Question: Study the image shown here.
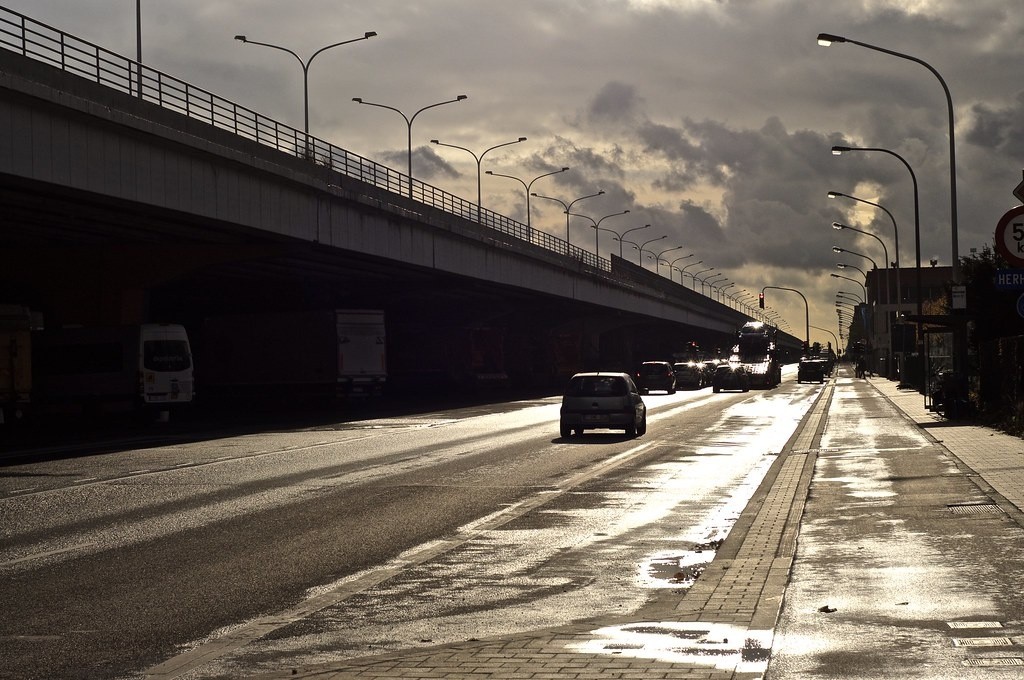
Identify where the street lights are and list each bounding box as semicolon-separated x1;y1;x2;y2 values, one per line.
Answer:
591;224;652;258
633;244;682;277
531;190;607;255
660;261;705;285
825;189;906;389
831;246;881;376
352;91;467;200
232;28;377;164
612;235;667;268
816;31;961;287
713;285;735;305
486;165;569;243
432;136;527;222
705;282;734;303
674;267;714;293
832;217;893;381
686;271;722;295
695;278;729;299
726;290;795;337
649;252;696;283
830;144;928;395
568;210;632;268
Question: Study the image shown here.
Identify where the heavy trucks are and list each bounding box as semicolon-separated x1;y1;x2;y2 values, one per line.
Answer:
729;336;782;389
190;305;390;406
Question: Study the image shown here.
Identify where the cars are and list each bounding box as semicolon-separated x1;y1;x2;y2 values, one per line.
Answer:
712;364;750;392
737;320;769;343
557;370;645;438
796;360;824;386
638;361;678;395
694;362;712;389
673;362;702;390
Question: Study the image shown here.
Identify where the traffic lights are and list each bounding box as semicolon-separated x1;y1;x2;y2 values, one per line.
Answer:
758;293;765;310
691;339;697;349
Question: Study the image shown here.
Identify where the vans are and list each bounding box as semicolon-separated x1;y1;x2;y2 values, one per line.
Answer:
38;323;194;423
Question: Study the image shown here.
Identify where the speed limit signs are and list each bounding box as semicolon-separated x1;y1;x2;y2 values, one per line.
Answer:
993;203;1024;269
